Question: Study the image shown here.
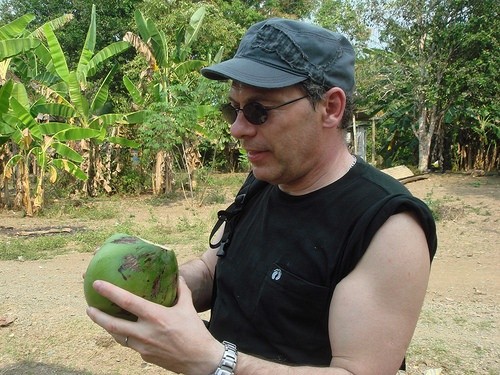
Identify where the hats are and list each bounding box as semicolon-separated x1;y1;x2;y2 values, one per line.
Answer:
202;18;356;96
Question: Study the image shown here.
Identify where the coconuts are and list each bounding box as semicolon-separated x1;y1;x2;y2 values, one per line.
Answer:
83;233;179;322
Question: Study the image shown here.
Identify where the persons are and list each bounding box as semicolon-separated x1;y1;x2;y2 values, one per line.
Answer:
82;17;438;375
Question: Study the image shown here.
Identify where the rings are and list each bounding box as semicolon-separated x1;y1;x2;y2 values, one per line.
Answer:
125;336;128;347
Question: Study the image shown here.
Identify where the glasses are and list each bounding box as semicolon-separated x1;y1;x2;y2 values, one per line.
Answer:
221;95;315;125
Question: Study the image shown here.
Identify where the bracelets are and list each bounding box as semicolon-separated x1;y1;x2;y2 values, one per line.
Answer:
210;341;238;375
